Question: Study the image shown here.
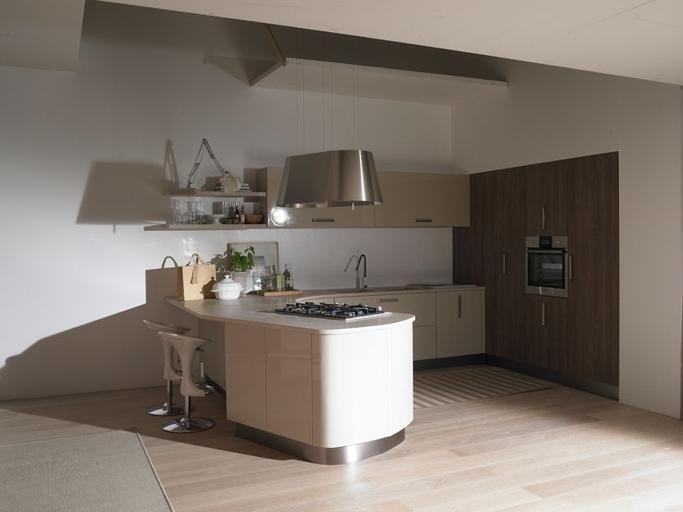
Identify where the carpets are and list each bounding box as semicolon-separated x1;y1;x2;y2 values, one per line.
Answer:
409;365;555;410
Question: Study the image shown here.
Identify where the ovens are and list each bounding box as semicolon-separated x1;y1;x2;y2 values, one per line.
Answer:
522;235;572;300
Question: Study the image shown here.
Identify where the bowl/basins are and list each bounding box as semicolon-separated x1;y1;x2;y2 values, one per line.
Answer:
245;214;264;224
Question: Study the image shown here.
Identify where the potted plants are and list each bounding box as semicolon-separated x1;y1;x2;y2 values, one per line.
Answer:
243;246;255;292
229;246;249;295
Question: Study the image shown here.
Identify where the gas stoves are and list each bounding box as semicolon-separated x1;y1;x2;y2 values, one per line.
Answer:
277;299;389;322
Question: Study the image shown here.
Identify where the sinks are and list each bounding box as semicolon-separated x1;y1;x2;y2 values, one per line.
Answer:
340;288;375;294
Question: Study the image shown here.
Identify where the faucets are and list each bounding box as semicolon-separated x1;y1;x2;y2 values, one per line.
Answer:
343;255;360;289
355;253;367;289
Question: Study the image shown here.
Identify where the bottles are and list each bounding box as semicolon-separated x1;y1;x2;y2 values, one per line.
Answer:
234;206;245;224
284;264;291;292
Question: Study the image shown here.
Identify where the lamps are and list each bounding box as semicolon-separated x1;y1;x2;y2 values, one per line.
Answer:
273;60;382;212
180;135;242;195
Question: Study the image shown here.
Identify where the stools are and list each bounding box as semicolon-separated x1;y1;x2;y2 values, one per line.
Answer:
141;318;217;434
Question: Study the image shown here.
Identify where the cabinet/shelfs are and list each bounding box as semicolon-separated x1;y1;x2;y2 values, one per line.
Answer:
562;152;619;401
525;295;566;372
525;162;561;235
162;190;267;229
372;170;472;229
434;291;485;359
334;293;436;361
256;166;374;228
451;167;526;364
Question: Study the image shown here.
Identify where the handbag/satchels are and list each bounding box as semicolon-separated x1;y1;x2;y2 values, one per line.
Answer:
175;253;217;301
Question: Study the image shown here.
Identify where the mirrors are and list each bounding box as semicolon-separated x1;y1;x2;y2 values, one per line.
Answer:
227;241;280;295
